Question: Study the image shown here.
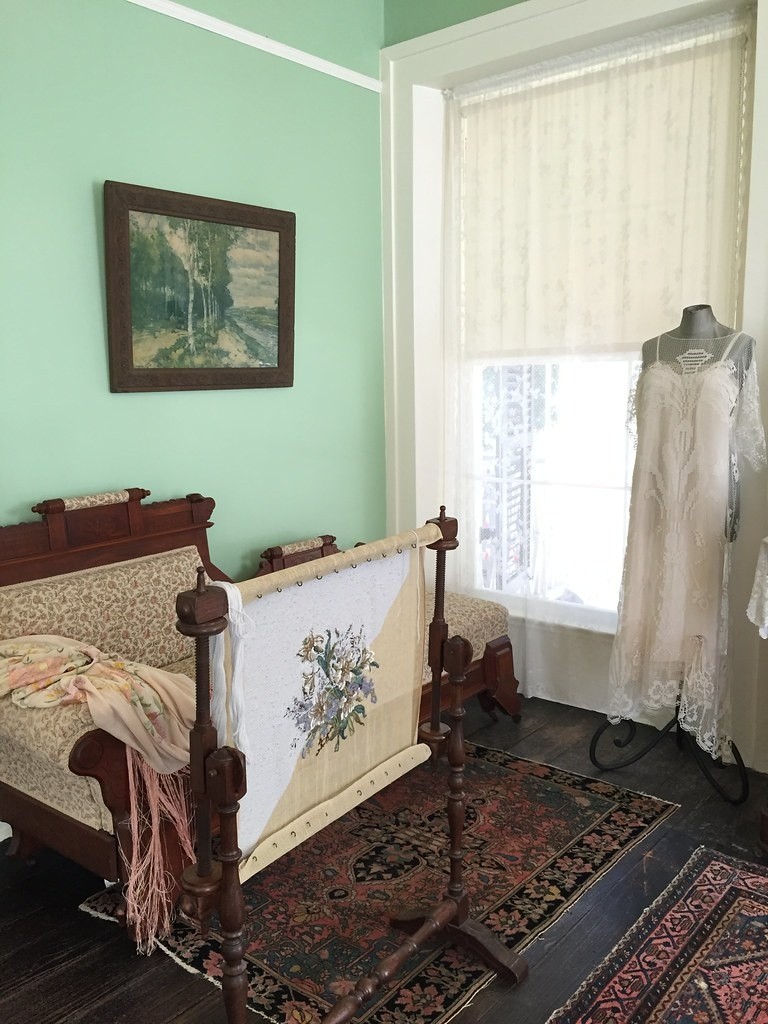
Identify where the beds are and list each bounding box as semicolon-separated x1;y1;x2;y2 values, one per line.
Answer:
0;487;529;1023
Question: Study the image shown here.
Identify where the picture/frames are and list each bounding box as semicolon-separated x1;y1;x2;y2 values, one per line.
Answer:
104;180;296;393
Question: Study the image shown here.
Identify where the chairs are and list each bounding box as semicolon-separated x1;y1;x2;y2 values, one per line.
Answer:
255;535;522;725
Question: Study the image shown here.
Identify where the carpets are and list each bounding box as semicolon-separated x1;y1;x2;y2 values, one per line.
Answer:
546;845;768;1024
80;739;681;1024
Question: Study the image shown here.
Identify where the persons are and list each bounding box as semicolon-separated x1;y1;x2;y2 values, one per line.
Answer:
609;304;765;764
